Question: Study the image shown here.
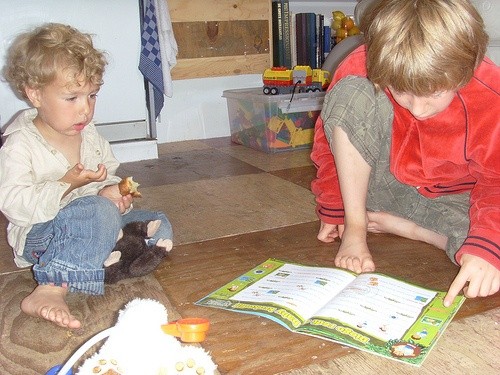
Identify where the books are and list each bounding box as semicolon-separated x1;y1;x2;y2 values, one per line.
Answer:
272;0;333;69
195;257;466;367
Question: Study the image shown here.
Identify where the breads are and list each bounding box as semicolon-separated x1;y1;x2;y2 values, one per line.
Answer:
118;175;142;198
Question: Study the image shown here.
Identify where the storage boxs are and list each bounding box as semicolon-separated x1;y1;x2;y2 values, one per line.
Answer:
221;87;326;153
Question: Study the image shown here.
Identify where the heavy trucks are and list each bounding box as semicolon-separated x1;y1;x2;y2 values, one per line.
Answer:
261;65;332;95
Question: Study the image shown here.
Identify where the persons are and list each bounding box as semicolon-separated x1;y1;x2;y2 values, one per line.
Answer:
310;0;500;306
0;21;173;330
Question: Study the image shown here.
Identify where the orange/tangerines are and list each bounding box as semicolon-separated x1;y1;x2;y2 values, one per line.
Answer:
332;11;360;43
